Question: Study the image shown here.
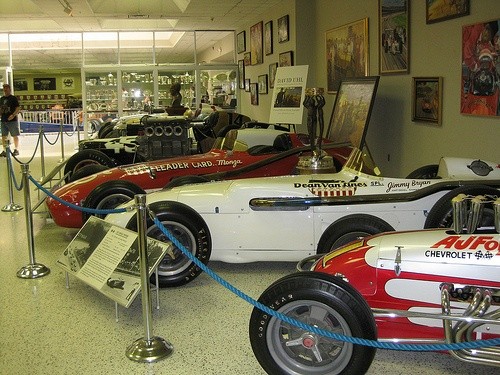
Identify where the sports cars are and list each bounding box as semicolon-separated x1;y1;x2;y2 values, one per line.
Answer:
104;146;500;290
44;127;360;243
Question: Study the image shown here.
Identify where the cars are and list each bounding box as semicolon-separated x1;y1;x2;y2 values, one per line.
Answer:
64;107;288;185
247;192;500;375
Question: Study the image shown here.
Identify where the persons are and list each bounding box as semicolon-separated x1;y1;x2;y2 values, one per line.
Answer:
302;94;326;138
169;81;182;107
0;84;20;157
275;88;285;107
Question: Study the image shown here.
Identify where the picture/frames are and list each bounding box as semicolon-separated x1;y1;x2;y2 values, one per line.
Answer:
250;21;263;65
249;83;258;105
279;51;293;67
245;79;250;92
277;15;289;43
326;76;381;158
237;31;246;54
265;20;273;56
238;60;245;89
325;17;370;93
426;0;470;25
258;74;268;94
378;0;410;75
269;62;278;88
244;52;251;65
412;76;442;125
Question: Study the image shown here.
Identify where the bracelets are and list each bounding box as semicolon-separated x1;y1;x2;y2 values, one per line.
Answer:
12;114;15;117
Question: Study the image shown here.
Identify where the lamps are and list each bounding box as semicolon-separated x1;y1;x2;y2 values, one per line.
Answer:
64;8;72;15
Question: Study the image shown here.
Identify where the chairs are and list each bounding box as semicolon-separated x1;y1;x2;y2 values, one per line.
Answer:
273;133;291;151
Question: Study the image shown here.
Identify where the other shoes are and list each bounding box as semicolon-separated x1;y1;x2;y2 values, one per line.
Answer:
13;149;19;155
0;151;6;157
